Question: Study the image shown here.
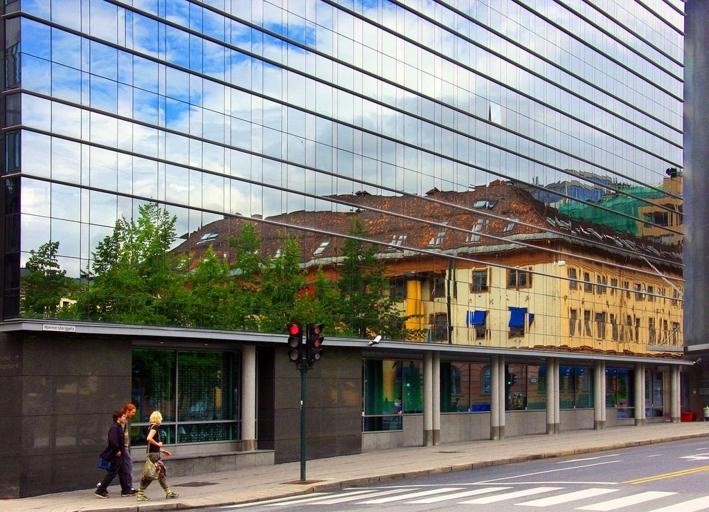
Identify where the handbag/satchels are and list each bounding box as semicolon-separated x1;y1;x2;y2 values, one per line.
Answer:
100;447;117;460
144;457;160;480
97;458;113;471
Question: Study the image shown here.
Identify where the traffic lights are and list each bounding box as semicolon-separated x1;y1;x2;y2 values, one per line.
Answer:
306;322;327;361
285;323;303;363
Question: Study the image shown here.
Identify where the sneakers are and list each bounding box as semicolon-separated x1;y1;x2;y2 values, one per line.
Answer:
95;489;110;499
121;490;137;497
138;495;150;502
166;492;179;499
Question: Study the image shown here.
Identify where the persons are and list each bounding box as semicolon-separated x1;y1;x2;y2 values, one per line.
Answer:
94;409;137;497
136;410;179;501
95;403;139;493
